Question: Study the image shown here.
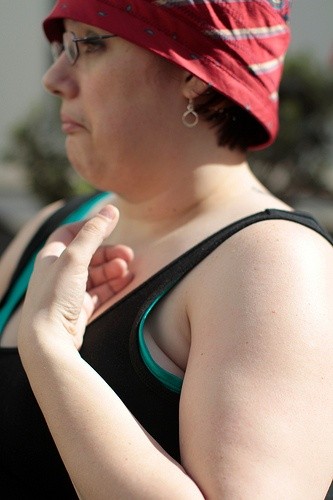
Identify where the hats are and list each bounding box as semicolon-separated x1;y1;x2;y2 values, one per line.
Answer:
43;0;290;151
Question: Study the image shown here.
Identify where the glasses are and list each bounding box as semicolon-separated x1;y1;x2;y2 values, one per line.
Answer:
51;32;118;65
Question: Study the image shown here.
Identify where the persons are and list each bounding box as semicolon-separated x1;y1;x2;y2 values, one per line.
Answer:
1;0;333;500
